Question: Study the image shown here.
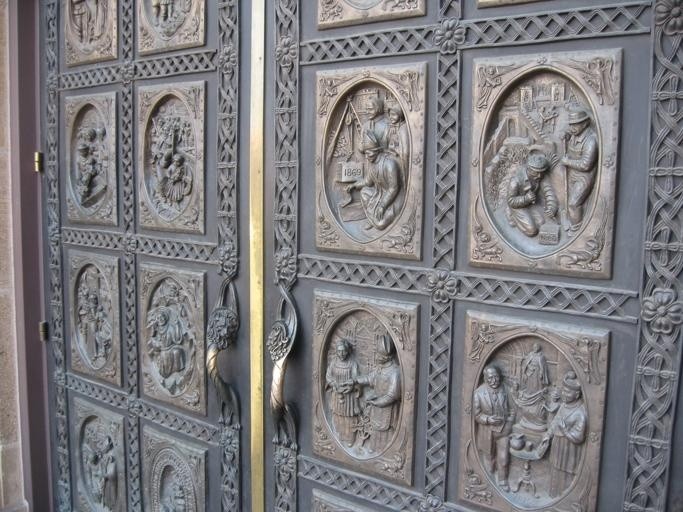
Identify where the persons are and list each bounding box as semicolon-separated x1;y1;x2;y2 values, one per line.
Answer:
76;1;194;512
325;100;600;512
